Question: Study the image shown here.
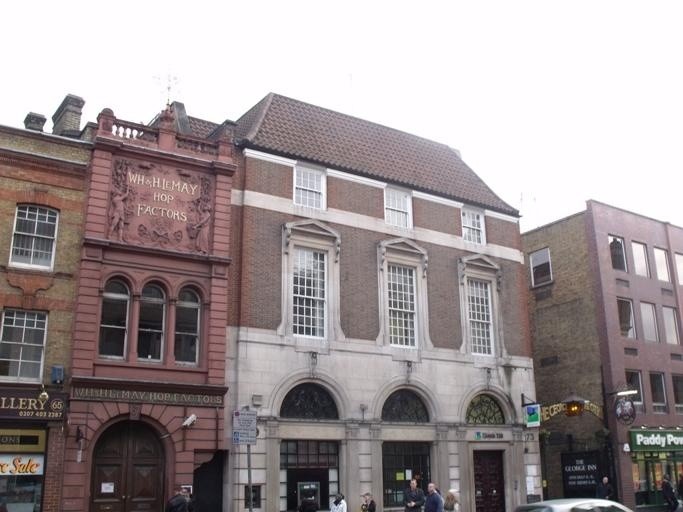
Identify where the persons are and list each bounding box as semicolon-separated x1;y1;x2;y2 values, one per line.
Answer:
402;478;425;512
165;485;188;512
183;488;200;512
329;493;348;512
660;473;679;512
424;482;444;512
298;491;320;512
106;182;131;245
191;199;212;256
595;476;615;500
444;493;462;512
362;492;376;512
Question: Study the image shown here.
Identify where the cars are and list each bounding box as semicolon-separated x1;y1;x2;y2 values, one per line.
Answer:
513;496;636;512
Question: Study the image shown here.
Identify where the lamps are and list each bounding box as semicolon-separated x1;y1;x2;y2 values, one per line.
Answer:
623;443;631;452
38;384;48;400
